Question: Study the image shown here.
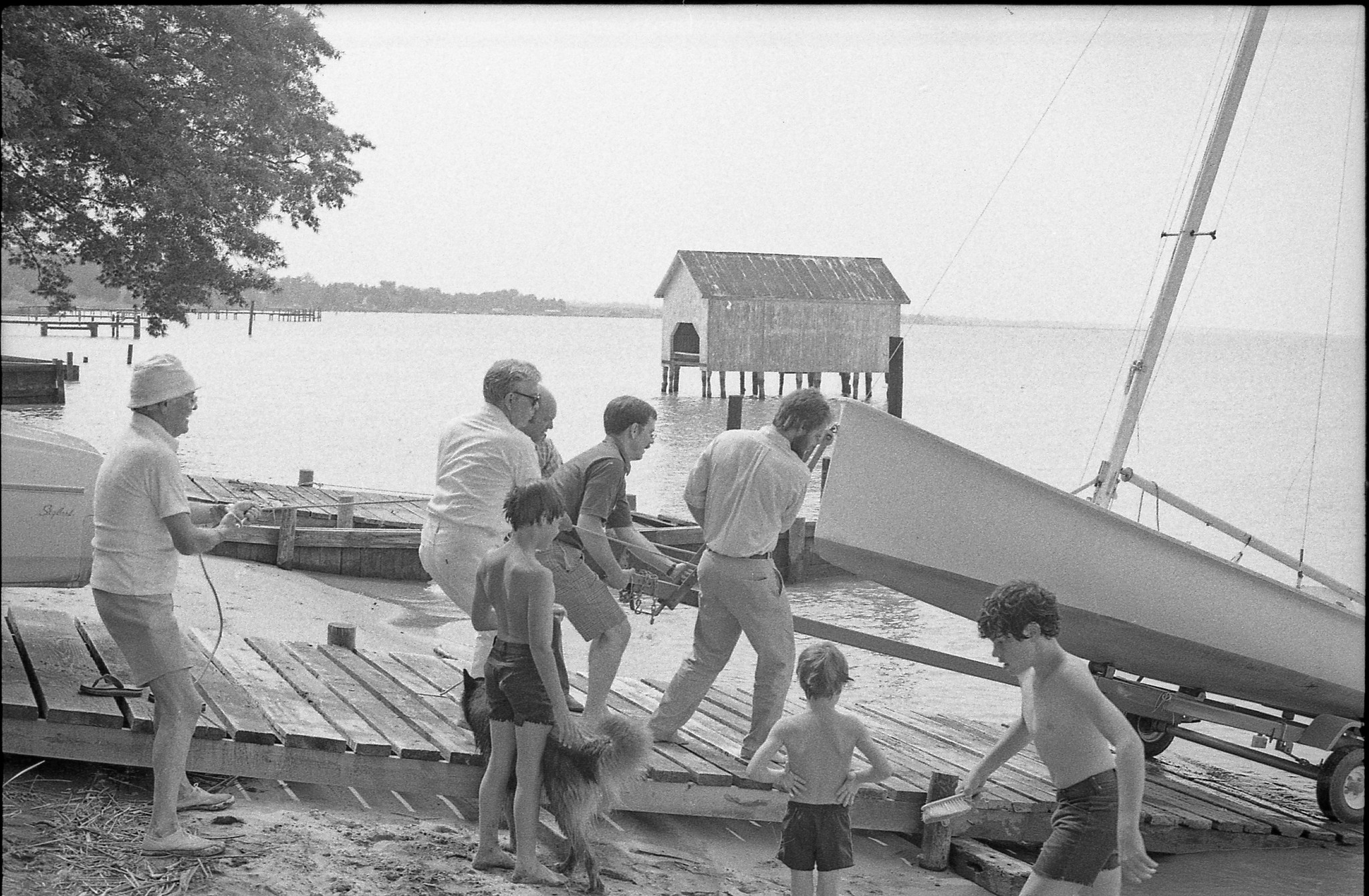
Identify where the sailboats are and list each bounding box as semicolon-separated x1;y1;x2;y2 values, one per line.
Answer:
806;6;1366;730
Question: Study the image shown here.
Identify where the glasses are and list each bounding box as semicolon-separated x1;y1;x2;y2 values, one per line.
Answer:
504;391;540;406
639;425;657;439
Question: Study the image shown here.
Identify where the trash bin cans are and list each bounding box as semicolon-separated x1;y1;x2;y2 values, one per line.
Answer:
1;418;104;587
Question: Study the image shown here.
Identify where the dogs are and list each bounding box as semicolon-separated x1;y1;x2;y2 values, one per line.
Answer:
460;666;658;896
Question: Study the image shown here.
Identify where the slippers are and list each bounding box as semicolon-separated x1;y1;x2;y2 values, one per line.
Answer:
80;675;142;697
147;692;207;711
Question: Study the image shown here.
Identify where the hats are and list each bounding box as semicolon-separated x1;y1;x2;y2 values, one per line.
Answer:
127;352;196;409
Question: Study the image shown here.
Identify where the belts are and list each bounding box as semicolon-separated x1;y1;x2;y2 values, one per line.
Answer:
706;546;773;559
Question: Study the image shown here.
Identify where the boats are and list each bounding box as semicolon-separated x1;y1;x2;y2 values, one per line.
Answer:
184;471;435;580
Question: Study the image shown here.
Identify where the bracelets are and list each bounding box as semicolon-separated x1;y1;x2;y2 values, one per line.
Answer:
666;562;677;576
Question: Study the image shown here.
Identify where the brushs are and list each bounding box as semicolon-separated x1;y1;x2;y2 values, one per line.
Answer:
920;787;990;824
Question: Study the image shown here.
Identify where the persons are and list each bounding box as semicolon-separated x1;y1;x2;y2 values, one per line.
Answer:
417;358;546;706
89;360;262;856
645;387;835;763
469;480;584;887
522;384;585;713
536;396;694;740
953;580;1160;896
740;640;894;896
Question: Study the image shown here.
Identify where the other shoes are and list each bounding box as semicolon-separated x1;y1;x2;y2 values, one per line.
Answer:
176;783;235;811
564;694;584;712
142;825;225;856
